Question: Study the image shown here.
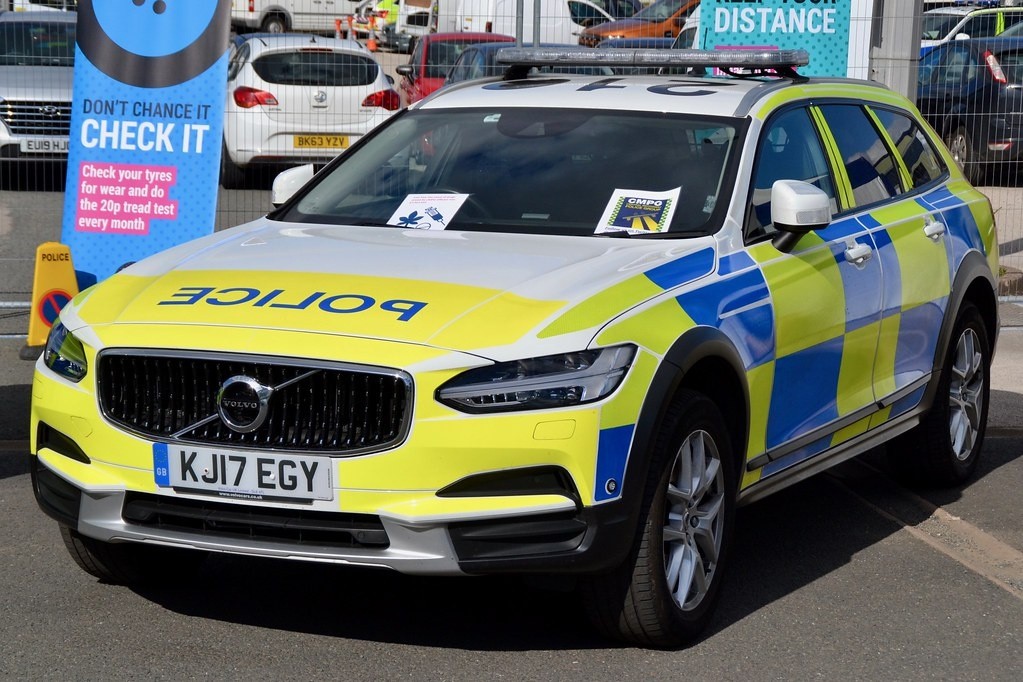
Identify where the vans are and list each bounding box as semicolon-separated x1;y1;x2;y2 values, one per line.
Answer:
455;0;615;54
396;0;457;49
231;1;362;34
658;4;701;75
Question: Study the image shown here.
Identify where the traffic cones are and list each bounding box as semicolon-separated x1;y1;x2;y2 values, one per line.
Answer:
336;16;380;52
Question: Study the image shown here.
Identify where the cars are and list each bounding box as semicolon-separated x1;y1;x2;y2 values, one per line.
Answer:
396;34;529;164
352;0;429;50
919;6;1023;184
31;50;1002;648
222;36;405;189
578;1;700;55
0;10;77;172
